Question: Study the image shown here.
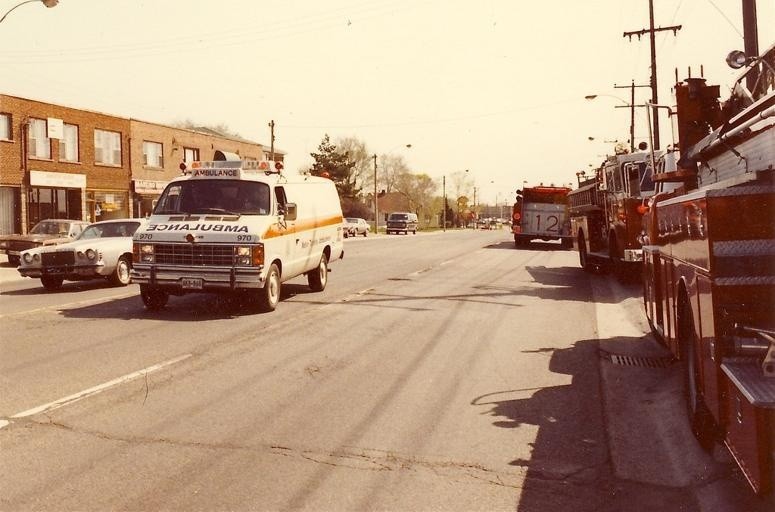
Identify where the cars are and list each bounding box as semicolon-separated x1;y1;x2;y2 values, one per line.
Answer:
346;217;371;237
0;219;93;264
342;218;350;239
19;218;148;288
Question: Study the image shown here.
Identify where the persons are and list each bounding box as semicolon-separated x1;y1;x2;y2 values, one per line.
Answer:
183;185;210;210
246;184;269;214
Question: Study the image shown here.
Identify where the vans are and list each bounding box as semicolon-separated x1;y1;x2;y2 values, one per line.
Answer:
386;212;419;234
129;159;345;309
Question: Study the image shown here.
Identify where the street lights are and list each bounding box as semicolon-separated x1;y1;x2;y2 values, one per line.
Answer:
0;0;59;23
